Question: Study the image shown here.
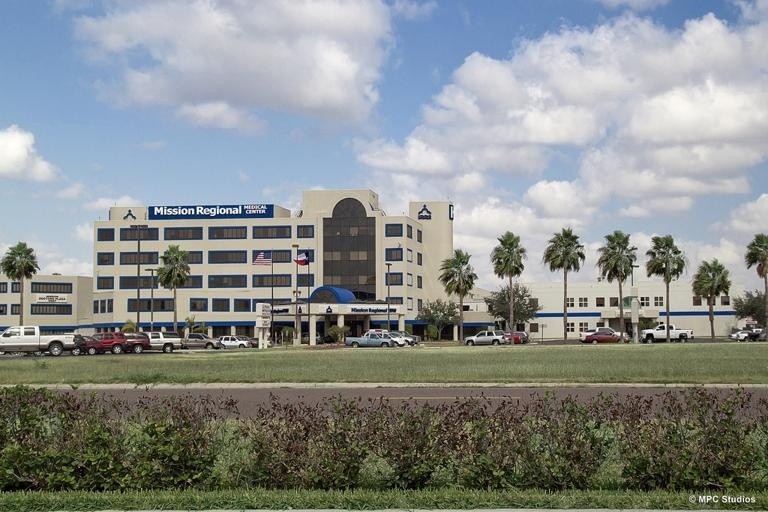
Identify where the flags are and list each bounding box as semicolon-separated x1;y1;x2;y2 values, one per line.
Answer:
252;251;272;266
293;250;313;266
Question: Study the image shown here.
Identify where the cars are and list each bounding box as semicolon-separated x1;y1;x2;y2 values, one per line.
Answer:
71;331;273;356
728;328;768;342
464;330;530;346
578;327;631;344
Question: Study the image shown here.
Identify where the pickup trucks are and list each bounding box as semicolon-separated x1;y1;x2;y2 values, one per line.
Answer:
0;325;75;357
638;324;694;344
345;328;422;348
